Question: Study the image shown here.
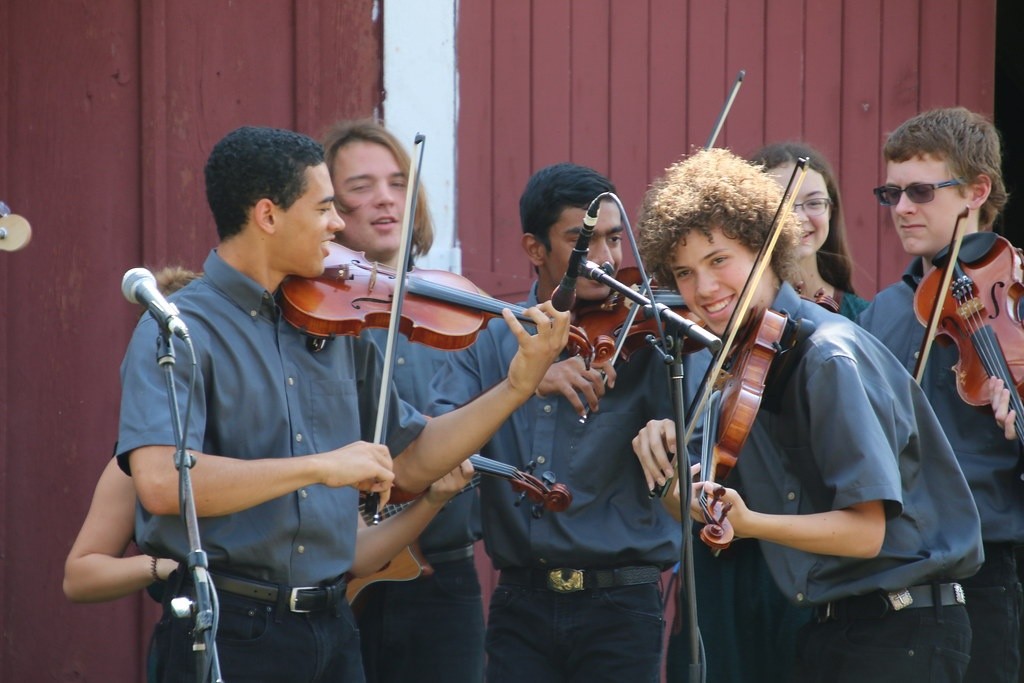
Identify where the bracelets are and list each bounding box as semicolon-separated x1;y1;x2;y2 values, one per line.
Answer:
535;388;548;400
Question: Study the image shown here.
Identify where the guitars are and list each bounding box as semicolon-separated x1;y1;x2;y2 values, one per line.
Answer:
344;468;487;608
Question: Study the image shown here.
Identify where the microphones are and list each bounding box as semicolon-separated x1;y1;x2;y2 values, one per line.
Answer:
121;267;190;340
552;200;600;312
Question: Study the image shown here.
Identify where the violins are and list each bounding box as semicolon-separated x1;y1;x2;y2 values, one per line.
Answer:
421;412;573;521
567;264;842;373
278;237;618;373
696;304;789;560
909;230;1024;483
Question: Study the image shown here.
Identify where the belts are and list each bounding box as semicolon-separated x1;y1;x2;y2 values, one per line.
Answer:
814;583;966;624
207;571;347;613
498;565;661;593
425;546;472;566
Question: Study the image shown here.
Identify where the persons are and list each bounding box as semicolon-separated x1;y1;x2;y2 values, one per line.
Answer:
117;120;571;683
633;143;990;683
63;122;880;683
857;99;1024;683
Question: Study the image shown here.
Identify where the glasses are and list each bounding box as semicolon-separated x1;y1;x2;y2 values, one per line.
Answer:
873;176;973;206
789;197;833;217
